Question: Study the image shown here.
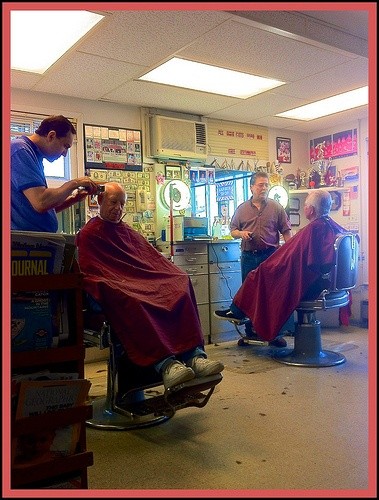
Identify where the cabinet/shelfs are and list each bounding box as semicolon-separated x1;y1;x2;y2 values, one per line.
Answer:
156;240;247;346
12;258;93;489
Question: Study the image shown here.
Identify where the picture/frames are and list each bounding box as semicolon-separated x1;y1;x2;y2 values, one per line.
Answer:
290;213;300;226
289;198;300;211
276;136;291;163
164;165;183;180
330;191;341;211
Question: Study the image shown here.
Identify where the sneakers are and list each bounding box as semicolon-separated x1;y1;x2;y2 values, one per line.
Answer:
182;355;224;377
214;309;241;321
161;360;195;388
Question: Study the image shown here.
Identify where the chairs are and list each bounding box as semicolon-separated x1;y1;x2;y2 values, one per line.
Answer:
230;233;360;368
80;290;222;431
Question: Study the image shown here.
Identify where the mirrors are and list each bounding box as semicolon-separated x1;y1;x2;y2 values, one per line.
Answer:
189;169;253;239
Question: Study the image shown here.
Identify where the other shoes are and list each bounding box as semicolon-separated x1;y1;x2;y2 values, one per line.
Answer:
238;338;249;346
269;338;287;347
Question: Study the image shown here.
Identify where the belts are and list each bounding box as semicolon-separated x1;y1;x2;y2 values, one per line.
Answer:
243;246;274;256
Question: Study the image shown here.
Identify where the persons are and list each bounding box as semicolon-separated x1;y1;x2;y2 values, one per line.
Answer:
77;181;224;386
215;189;350;341
230;171;292;348
10;115;100;232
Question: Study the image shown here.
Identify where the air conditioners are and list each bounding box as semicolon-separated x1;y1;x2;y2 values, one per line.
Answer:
150;116;208;159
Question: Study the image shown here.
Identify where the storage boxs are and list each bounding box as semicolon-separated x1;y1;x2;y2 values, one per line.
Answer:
166;217;209;242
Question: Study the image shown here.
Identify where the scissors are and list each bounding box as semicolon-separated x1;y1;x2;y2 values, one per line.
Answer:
248;233;253;241
